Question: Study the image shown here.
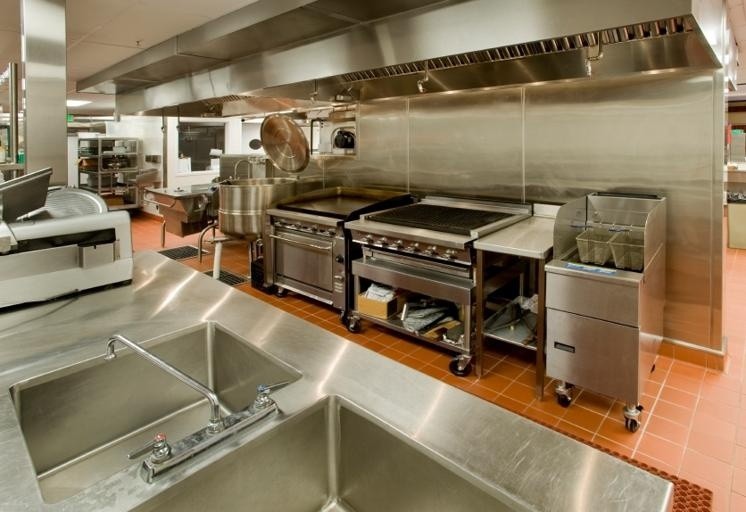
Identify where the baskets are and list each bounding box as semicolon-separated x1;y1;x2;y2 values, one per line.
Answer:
576;227;644;270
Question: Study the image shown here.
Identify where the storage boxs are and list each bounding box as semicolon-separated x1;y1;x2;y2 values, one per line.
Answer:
101;195;124;205
77;158;98;171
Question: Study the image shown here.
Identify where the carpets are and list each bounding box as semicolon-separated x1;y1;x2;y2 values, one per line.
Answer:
518;414;712;512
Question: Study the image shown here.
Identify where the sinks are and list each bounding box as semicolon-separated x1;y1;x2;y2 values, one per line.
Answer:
129;393;534;512
10;323;303;505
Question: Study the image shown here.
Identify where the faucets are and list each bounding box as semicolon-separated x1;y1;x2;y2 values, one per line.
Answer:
104;335;222;423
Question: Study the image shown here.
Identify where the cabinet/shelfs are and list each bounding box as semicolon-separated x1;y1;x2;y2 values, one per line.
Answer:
77;138;140;210
